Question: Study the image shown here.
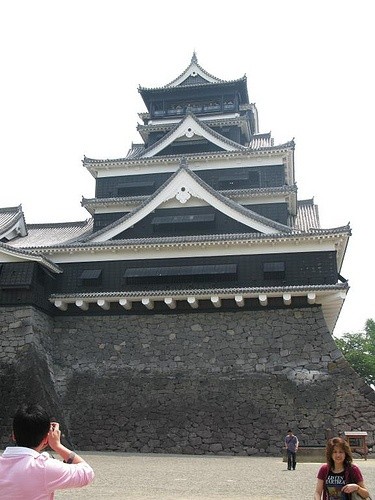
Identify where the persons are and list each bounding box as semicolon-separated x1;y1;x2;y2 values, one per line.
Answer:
313;438;371;500
0;402;94;500
284;429;299;470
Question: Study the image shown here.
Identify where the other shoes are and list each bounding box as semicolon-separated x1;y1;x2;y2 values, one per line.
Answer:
293;467;295;470
288;467;291;470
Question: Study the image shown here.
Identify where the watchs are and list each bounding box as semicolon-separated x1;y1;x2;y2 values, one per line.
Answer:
356;484;360;491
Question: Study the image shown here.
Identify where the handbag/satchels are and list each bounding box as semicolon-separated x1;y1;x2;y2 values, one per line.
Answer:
347;466;367;499
